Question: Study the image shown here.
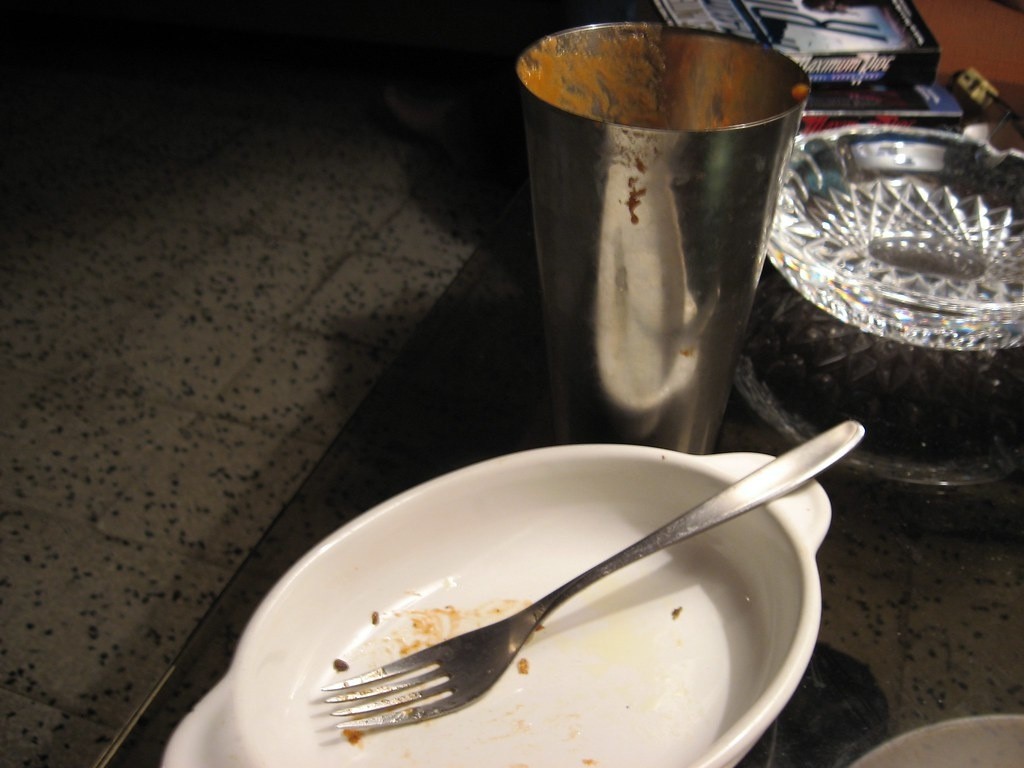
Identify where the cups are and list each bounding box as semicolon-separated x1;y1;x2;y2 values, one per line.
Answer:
513;20;811;460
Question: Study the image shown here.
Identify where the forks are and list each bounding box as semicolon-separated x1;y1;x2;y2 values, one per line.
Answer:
317;418;869;738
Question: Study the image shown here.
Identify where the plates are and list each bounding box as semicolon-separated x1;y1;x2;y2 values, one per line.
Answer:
765;120;1024;351
158;440;833;768
847;709;1024;768
